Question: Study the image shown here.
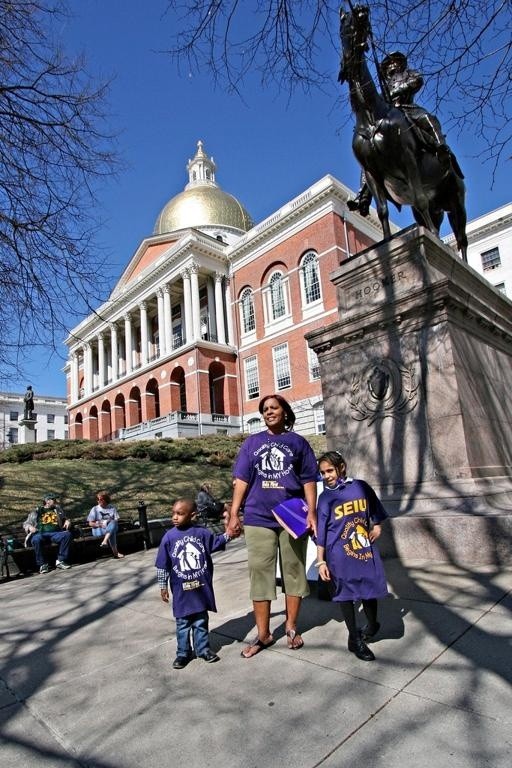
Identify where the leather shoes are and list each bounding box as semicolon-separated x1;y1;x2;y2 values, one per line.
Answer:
172;654;193;669
200;650;219;663
348;637;374;661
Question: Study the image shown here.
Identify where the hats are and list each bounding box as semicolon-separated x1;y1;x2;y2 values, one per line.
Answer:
42;492;59;500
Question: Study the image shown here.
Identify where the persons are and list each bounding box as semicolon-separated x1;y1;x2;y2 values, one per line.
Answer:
316;451;388;661
347;51;456;217
155;496;241;668
228;393;319;658
197;483;230;534
20;385;34;420
86;490;125;559
23;492;72;574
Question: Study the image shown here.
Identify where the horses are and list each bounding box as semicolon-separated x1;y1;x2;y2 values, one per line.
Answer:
337;0;469;264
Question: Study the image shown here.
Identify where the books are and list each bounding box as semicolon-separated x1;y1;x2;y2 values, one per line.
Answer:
270;497;310;541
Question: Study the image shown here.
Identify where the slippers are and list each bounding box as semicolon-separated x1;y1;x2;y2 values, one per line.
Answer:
284;628;304;650
240;636;276;658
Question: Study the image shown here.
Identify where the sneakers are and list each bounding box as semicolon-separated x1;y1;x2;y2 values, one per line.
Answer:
55;559;72;570
360;622;381;641
99;542;108;548
40;562;49;574
114;553;125;559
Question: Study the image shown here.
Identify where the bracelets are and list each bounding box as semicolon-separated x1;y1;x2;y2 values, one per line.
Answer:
315;561;328;568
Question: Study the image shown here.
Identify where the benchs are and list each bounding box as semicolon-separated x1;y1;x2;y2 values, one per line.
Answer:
193;500;245;534
1;513;146;581
